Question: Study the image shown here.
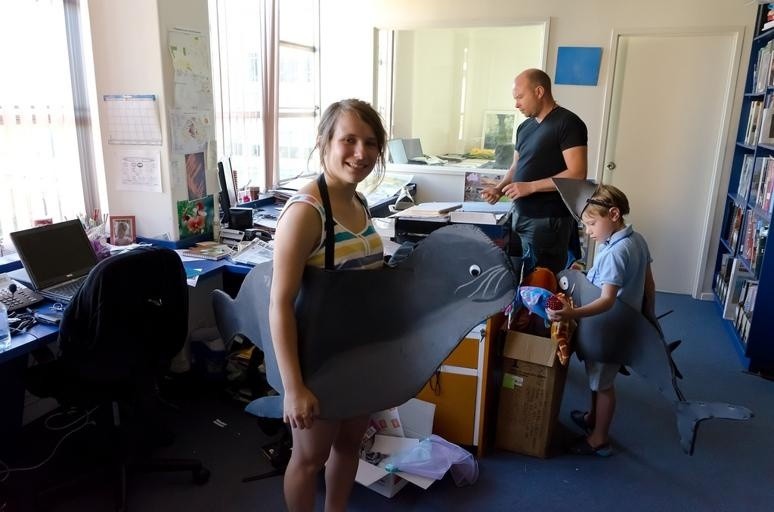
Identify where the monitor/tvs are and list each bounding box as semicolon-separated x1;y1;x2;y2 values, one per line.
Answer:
218;154;238;224
387;138;409;164
403;139;424;160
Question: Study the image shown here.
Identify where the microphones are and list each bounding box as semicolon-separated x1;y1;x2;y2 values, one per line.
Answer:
6;284;17;312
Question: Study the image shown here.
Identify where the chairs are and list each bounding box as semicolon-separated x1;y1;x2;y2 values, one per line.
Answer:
22;245;210;508
489;144;516;169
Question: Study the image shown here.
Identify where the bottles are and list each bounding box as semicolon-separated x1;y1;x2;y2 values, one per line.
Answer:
0;301;12;354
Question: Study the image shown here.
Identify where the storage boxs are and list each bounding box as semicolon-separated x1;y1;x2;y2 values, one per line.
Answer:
355;394;439;499
496;308;575;461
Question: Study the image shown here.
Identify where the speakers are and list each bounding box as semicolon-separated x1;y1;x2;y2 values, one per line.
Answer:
228;207;254;230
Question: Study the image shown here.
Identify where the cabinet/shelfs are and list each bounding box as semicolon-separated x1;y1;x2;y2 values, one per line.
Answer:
394;308;506;461
711;2;772;379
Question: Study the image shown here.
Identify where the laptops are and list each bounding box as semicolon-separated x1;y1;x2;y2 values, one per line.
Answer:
10;219;100;305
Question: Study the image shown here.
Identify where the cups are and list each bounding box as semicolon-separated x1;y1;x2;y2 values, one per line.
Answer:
249;187;259;201
34;217;52;227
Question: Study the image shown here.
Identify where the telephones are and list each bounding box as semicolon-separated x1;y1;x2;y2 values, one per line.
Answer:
242;228;272;241
0;273;45;315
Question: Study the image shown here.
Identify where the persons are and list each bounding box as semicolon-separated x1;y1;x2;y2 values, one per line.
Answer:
115;223;132;245
546;183;655;454
478;69;587;281
269;98;388;511
194;204;206;216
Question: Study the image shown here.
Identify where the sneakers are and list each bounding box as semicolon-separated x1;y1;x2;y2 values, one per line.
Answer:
566;436;614;458
570;408;595;433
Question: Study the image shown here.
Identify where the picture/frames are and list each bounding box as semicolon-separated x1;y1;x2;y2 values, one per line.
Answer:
480;110;518;151
110;216;135;245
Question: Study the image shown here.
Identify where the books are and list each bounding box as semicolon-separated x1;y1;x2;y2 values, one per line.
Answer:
409;202;463;215
711;4;774;350
183;245;230;262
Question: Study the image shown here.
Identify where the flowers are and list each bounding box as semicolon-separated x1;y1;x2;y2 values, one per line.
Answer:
183;203;206;234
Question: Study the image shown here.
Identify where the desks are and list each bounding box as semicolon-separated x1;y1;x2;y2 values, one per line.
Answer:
440;155;495;168
222;178;528;464
0;248;227;449
